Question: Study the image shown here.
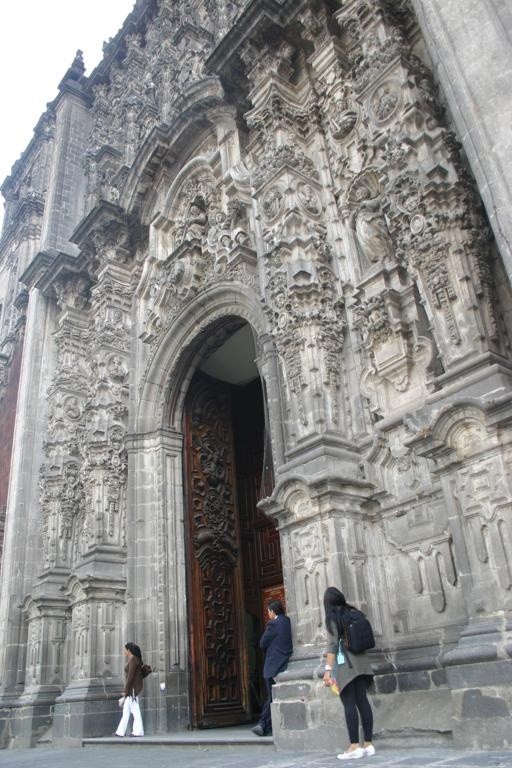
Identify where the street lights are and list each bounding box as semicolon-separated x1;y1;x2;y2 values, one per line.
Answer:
142;664;152;678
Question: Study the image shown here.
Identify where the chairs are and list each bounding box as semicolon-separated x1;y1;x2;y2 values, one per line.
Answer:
113;733;141;737
252;725;272;736
337;744;376;759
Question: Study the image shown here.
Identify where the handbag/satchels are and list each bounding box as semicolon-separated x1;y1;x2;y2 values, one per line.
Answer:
324;664;333;671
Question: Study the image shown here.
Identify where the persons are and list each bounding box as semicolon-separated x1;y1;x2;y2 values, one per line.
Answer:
321;586;376;760
112;642;146;738
183;204;207;242
250;600;293;738
339;184;394;269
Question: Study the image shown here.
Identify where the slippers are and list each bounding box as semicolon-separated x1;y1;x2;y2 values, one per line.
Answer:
341;610;375;653
